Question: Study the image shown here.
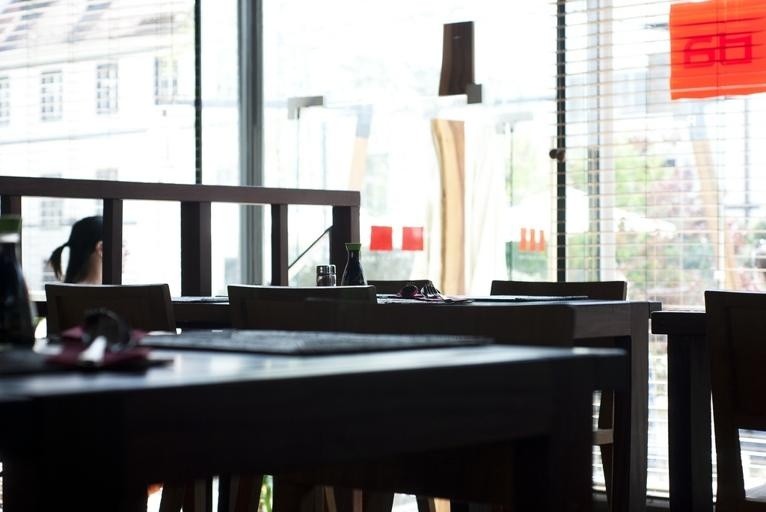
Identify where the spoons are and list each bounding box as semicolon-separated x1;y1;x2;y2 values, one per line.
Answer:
76;308;132;368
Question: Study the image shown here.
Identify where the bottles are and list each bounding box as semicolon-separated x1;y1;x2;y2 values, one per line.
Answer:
0;234;33;344
316;242;366;286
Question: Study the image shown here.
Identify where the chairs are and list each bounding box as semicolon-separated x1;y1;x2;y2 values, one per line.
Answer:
43;283;175;338
651;291;765;510
491;276;629;495
228;285;436;509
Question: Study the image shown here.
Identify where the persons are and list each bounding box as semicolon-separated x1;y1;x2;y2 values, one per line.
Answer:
35;215;104;340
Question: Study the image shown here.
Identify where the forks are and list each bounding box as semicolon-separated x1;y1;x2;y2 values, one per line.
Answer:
422;279;454;301
